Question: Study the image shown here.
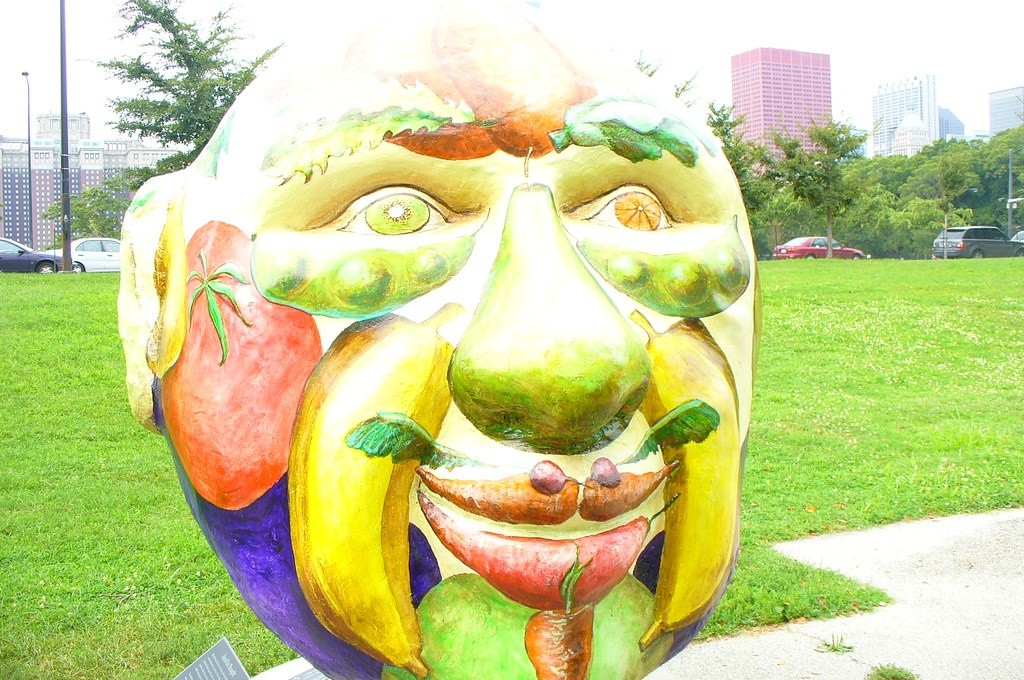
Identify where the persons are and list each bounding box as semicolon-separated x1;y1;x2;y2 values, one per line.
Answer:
117;28;759;680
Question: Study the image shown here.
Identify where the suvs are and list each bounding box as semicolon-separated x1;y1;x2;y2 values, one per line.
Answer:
932;225;1024;260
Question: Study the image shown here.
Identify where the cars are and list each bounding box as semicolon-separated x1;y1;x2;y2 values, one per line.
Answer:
46;237;121;273
0;236;63;275
772;236;866;260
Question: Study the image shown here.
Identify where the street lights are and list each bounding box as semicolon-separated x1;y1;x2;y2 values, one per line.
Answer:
21;71;35;248
1006;147;1023;240
